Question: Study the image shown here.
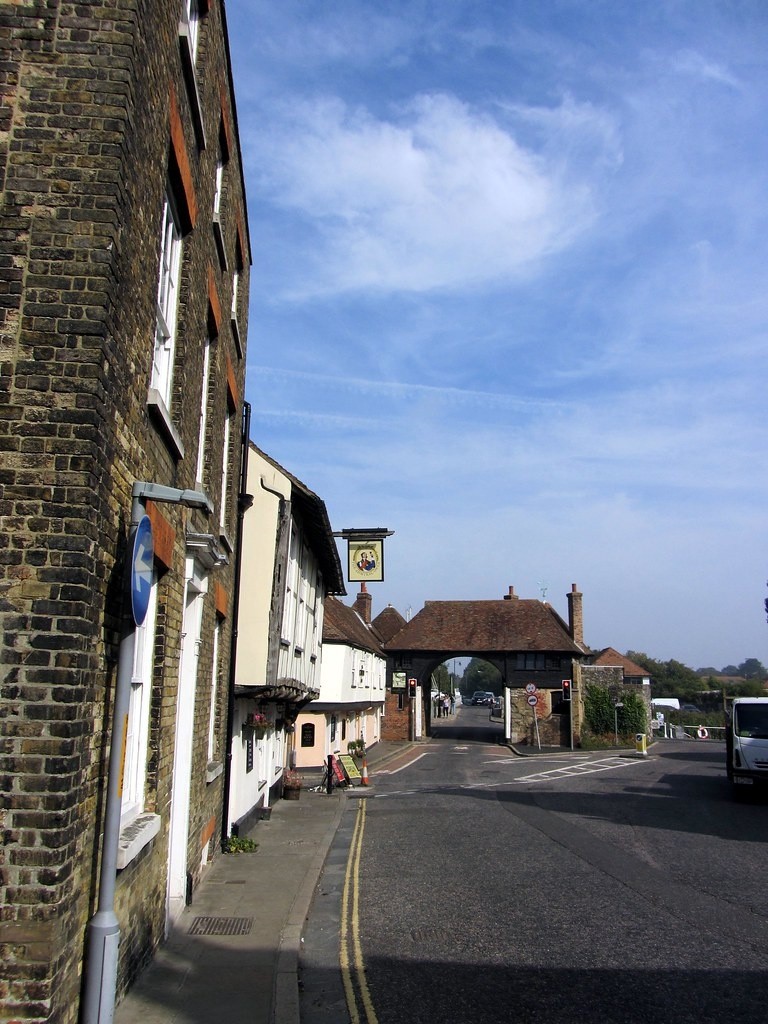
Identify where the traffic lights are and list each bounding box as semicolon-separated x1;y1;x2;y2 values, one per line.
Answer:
562;680;572;701
409;679;417;698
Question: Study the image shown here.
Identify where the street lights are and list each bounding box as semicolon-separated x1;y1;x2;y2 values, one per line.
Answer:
437;665;442;718
452;659;461;698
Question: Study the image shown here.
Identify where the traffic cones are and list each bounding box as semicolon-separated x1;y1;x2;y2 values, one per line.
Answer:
361;760;369;787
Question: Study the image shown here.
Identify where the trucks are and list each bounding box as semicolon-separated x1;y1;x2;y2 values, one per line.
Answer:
723;696;768;777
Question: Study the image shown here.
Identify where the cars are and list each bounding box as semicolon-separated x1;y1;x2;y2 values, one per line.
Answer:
486;691;495;704
472;691;488;706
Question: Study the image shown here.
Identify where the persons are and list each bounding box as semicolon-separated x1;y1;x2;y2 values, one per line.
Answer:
490;696;496;709
450;695;456;715
443;696;449;717
